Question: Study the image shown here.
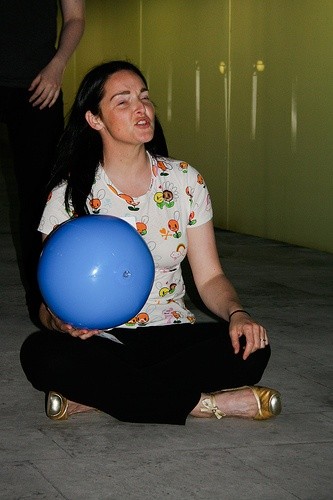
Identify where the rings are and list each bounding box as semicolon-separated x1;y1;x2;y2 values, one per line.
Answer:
260;339;266;342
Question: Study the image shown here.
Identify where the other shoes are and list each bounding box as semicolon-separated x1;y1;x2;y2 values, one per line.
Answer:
47;392;67;419
206;386;280;421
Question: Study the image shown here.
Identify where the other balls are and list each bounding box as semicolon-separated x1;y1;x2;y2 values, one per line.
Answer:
36;213;157;332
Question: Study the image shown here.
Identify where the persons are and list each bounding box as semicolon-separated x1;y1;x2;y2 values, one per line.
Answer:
20;61;281;425
0;0;85;332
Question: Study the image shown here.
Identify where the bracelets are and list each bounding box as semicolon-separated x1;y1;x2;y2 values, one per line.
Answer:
229;310;252;322
